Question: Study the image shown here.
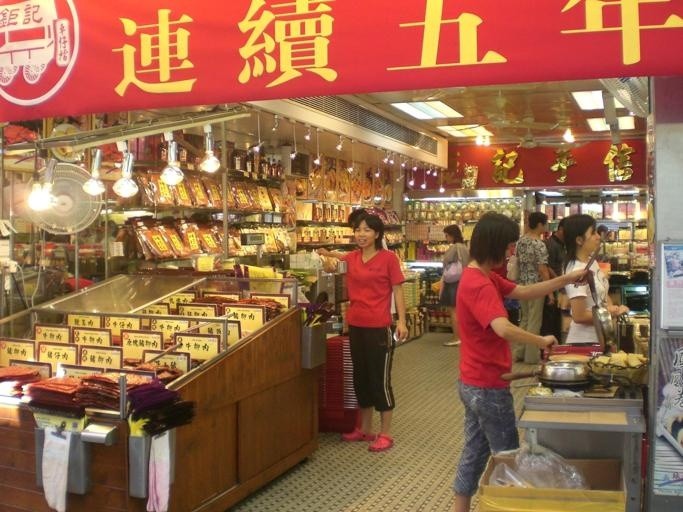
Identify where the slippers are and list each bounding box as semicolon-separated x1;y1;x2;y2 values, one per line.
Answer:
444;340;461;346
341;428;376;441
368;434;394;451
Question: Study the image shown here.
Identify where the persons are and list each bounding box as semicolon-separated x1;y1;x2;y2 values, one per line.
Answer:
329;213;405;452
543;212;629;348
512;211;556;364
319;208;397;324
455;211;591;512
441;225;469;346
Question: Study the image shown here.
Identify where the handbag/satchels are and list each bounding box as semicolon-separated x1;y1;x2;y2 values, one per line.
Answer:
443;261;463;283
506;256;520;281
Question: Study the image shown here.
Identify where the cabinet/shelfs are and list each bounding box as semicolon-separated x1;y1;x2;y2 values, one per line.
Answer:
297;219;406;248
0;308;318;512
126;205;292;263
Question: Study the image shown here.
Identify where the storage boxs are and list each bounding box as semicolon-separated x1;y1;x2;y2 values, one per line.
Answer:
478;452;628;512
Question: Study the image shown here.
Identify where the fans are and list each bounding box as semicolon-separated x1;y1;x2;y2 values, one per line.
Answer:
516;93;580;149
25;164;102;292
457;85;560;132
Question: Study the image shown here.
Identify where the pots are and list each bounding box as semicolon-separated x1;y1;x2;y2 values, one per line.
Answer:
584;269;616;354
500;359;592;389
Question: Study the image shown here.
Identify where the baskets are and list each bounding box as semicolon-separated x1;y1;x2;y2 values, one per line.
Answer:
587;355;648;383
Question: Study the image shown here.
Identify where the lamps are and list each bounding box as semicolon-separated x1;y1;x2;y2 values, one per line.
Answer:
254;106;446;194
28;111;252;212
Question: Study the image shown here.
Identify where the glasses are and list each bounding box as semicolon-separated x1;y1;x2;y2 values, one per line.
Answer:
509;245;516;254
